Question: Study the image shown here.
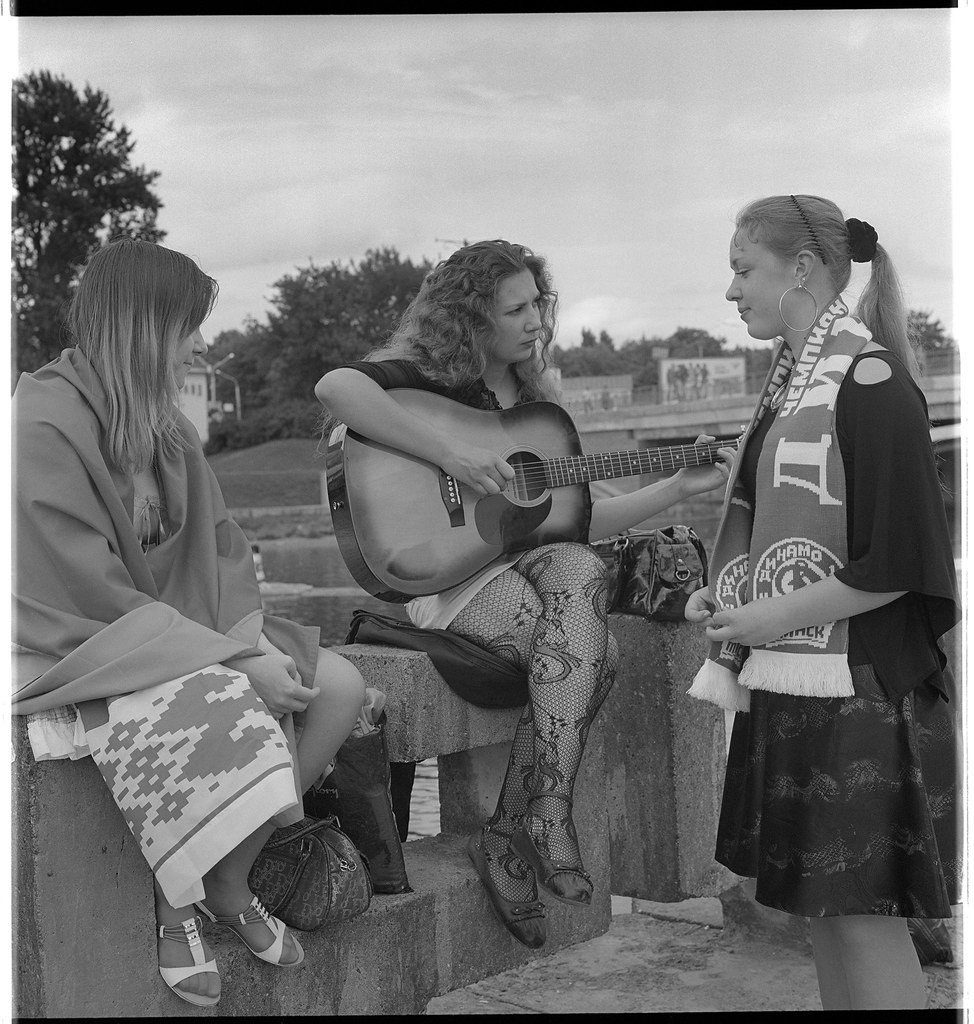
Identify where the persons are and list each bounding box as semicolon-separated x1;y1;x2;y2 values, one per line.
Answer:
686;194;962;1010
315;239;738;953
12;242;366;1007
583;361;711;417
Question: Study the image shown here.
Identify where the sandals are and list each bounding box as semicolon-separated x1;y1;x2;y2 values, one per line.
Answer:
195;894;305;967
157;914;221;1007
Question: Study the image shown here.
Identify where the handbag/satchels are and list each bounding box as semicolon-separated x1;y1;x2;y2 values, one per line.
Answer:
247;813;374;931
303;726;414;894
345;609;530;707
589;524;708;620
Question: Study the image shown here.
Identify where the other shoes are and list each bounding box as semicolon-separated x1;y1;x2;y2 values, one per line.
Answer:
467;821;546;949
509;790;594;905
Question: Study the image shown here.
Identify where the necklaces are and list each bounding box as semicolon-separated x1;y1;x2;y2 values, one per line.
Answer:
770;366;793;412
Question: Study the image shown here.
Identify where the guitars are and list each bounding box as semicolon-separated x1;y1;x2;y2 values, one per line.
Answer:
323;388;748;604
